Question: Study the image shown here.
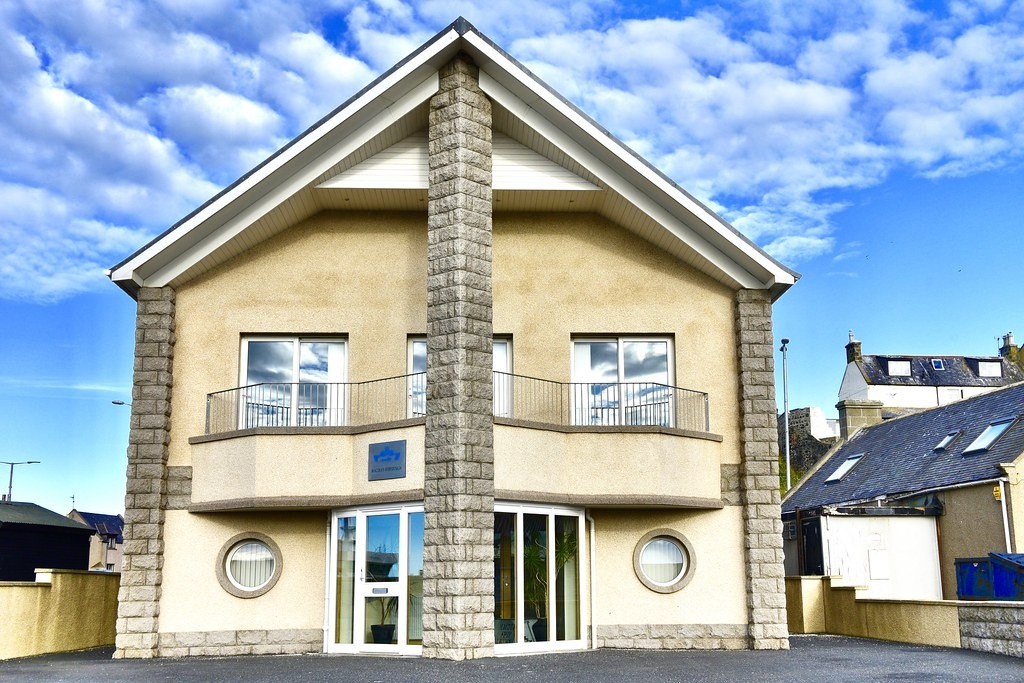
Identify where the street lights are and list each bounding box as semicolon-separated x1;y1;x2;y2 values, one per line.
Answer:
779;337;791;490
0;460;40;500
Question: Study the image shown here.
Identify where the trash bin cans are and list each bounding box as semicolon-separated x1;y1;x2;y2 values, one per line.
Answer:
988;551;1024;602
954;557;993;601
